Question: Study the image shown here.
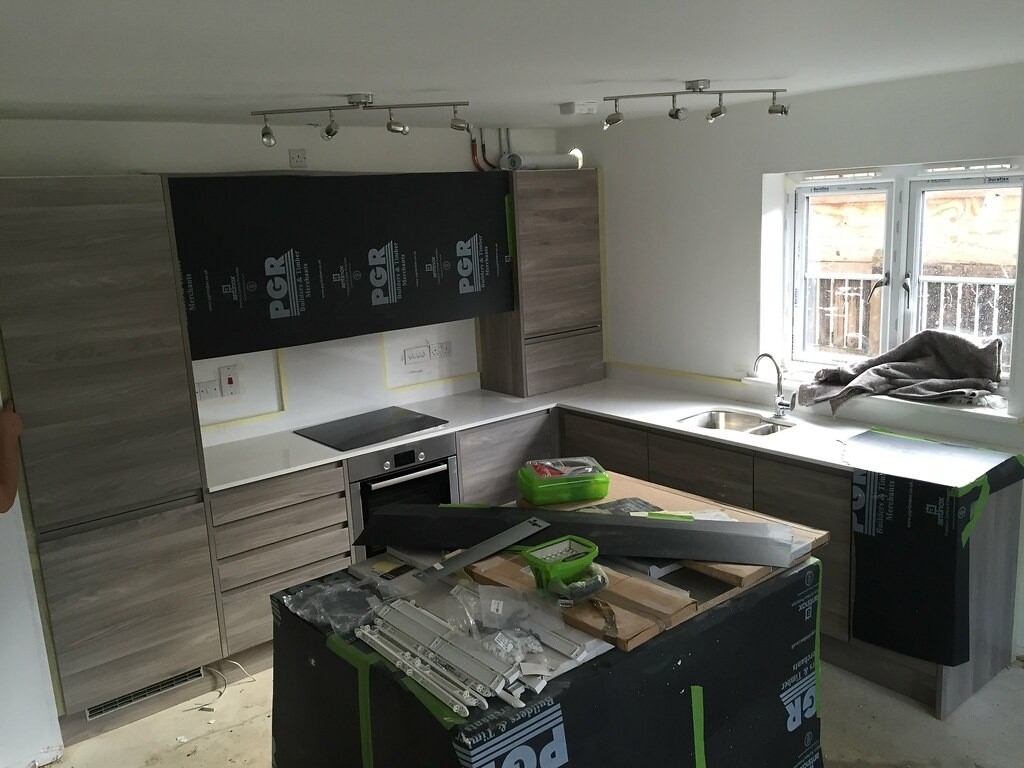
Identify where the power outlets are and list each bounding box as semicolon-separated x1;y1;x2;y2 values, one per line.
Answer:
194;380;219;400
289;148;307;168
430;343;441;358
441;341;453;356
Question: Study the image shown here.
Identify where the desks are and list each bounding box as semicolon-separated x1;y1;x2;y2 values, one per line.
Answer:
271;470;831;768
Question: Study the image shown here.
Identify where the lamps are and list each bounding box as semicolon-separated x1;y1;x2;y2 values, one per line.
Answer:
601;99;623;130
451;105;473;134
387;108;410;135
768;92;790;116
321;111;339;140
706;93;727;123
260;114;276;147
668;95;689;121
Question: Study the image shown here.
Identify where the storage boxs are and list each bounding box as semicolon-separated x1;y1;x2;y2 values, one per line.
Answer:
517;456;610;505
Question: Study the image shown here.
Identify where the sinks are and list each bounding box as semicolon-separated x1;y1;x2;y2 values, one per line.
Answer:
749;423;796;436
676;407;770;434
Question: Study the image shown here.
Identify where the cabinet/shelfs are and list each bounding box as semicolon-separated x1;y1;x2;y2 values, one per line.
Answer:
0;167;1024;768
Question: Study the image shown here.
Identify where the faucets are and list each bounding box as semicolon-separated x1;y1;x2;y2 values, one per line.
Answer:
751;352;797;420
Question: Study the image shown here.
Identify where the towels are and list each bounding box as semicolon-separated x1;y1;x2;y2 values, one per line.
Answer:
797;327;1003;412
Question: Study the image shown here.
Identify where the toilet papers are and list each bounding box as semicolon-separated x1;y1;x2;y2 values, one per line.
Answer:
498;153;580;171
